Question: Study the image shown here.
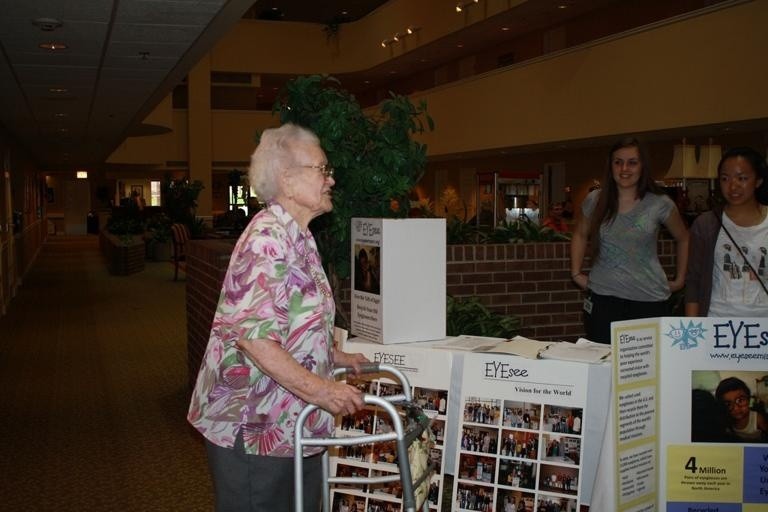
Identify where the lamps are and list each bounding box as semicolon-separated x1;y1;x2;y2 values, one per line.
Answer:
381;25;421;48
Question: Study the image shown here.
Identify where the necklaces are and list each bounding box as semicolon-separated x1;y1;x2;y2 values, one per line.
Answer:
306;254;330;296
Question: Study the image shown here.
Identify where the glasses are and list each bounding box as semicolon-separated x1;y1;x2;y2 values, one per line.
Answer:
724;398;747;410
301;165;335;177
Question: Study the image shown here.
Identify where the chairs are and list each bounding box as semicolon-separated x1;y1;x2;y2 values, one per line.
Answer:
170;221;193;281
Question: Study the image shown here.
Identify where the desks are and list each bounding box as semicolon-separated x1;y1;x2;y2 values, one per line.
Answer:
47;212;65;236
323;335;614;512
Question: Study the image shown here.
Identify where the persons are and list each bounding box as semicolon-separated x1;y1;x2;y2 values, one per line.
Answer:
570;139;688;345
336;380;581;512
186;124;370;512
684;147;768;318
719;383;767;443
542;200;569;234
355;248;381;295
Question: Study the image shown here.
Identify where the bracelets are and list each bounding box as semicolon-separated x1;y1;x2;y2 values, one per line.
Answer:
571;271;581;279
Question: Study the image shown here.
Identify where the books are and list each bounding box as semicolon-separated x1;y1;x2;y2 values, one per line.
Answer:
436;330;616;365
